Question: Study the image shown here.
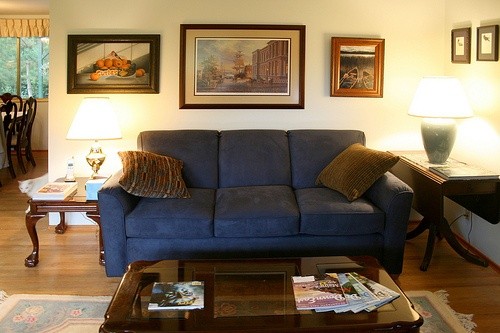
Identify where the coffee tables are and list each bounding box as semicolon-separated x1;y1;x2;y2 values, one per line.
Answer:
99;255;424;333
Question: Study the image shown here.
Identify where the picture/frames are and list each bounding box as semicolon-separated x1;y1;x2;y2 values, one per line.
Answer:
330;37;385;98
477;25;498;61
451;27;471;64
179;24;306;109
67;34;159;94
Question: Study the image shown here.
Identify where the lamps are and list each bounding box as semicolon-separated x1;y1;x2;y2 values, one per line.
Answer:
407;76;474;163
65;97;122;185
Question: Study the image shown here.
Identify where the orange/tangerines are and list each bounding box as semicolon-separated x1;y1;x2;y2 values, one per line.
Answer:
90;59;145;81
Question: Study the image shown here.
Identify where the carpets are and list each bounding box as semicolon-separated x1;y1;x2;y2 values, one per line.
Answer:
0;290;477;333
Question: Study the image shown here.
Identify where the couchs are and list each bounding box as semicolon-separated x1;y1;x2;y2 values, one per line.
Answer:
97;130;414;277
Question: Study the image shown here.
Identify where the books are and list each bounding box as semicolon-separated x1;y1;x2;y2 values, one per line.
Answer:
429;165;500;180
32;182;78;200
148;281;205;310
291;272;400;313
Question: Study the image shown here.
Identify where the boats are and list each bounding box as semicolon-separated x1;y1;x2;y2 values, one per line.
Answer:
340;67;359;89
362;70;374;88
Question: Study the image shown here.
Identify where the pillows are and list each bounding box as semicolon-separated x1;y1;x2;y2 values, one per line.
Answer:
117;151;191;199
315;142;400;202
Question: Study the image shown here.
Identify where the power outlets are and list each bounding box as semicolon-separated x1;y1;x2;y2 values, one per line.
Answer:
464;209;471;220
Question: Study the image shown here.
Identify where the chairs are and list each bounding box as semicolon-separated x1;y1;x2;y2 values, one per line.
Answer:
0;93;37;187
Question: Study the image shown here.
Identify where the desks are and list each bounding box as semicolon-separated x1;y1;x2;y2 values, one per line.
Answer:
387;150;500;271
25;176;110;268
0;111;29;169
